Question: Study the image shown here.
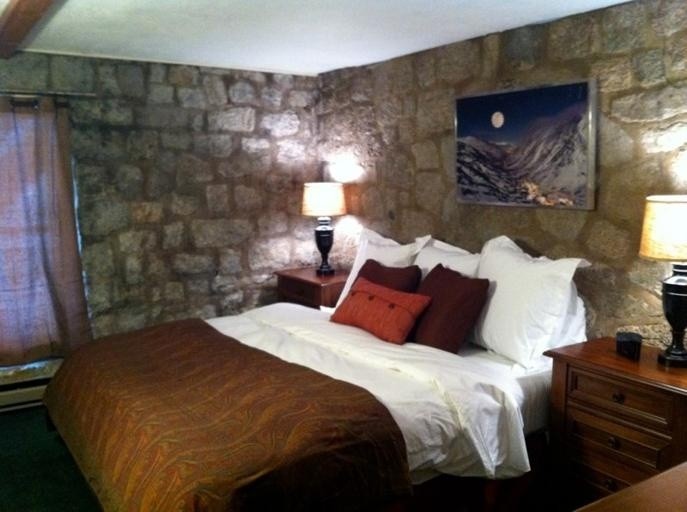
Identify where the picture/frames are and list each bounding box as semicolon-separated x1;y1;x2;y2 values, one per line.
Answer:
452;76;597;212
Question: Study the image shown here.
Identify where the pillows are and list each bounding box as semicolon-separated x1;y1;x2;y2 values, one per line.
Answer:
329;228;591;370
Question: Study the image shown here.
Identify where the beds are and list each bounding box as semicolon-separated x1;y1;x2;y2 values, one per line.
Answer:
43;227;588;512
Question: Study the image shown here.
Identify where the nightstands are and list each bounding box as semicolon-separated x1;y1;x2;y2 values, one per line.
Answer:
543;336;687;506
275;267;350;310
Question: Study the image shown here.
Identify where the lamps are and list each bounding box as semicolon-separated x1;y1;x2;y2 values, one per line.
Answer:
301;182;348;276
639;194;687;368
